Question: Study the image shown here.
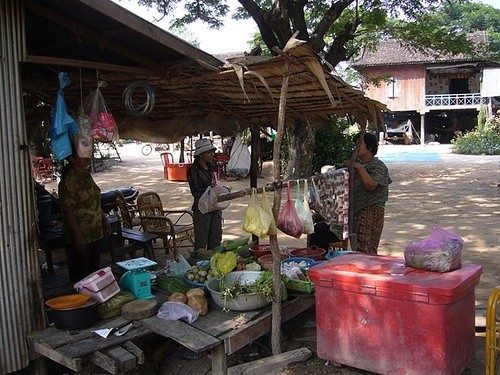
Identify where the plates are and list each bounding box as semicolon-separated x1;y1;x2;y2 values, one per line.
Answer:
46;294;91;308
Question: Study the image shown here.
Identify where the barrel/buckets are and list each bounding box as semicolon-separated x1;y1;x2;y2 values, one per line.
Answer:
35;198;52;226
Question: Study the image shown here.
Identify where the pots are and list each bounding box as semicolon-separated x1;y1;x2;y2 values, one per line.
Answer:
47;298;104;331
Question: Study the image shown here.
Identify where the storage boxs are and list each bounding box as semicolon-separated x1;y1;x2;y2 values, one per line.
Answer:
306;253;484;375
73;266;121;309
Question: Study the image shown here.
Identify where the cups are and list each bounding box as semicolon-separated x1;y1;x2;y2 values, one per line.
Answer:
389;257;406;277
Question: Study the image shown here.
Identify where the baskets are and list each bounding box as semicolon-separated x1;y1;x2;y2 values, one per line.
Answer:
283;267;315;293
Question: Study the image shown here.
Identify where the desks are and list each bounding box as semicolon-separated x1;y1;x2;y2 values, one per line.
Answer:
37;213;125;276
166;163;194;182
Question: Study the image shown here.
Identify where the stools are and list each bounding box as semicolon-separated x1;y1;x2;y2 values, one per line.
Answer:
112;228;159;261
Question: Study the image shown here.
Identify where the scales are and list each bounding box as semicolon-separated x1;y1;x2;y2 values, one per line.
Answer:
115;256;159;300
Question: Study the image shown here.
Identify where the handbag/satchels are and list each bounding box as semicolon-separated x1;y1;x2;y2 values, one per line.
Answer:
403;226;462;272
243;180;315;237
225;135;251;178
198;172;231;214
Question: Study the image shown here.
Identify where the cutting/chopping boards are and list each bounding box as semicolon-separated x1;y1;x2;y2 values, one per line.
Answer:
121;298;158;320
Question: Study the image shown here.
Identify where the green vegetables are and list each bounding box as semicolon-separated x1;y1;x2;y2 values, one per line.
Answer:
154;274;190;294
405;239;462;273
217;270;289;313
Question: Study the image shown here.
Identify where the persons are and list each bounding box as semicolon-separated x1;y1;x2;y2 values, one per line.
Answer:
186;139;222;252
58;155;112;283
224;137;236;156
343;131;392;255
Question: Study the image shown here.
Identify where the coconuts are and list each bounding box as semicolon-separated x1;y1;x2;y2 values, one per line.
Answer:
168;288;208;316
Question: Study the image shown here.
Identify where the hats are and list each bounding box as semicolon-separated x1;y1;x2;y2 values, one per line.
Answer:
194;139;217;156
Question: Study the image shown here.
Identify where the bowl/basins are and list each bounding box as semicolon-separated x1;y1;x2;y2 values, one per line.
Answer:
183;269;207;288
206;271;272;311
249;245;359;272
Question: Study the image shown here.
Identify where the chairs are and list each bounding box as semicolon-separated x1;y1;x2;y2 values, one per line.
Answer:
32;157;55;182
114;190;157;244
485;285;500;375
160;152;173;180
137;192;196;262
